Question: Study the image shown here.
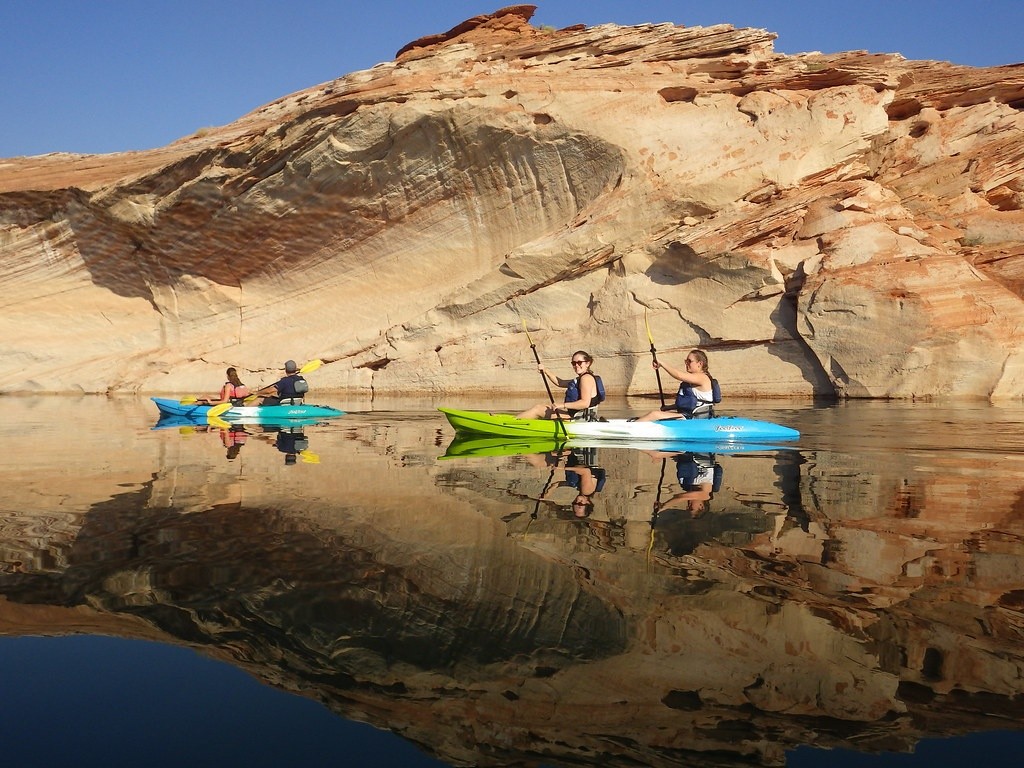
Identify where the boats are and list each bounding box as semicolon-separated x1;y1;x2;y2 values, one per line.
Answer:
148;396;347;418
436;434;802;462
436;406;803;445
150;413;319;433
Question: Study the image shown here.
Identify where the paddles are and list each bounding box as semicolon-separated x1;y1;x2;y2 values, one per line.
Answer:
640;308;666;408
207;360;322;416
206;422;320;466
523;449;565;535
520;320;573;443
179;396;221;405
647;454;669;558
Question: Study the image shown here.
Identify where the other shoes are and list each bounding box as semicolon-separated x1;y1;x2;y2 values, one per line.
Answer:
599;416;608;422
489;412;494;416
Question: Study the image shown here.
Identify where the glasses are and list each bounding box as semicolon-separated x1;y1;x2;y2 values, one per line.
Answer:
571;360;588;366
686;506;696;511
684;359;697;365
572;501;588;507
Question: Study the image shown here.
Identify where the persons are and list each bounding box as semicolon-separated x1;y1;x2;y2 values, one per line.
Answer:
635;450;724;519
197;424;247;459
524;448;606;518
244;359;308;407
253;425;308;466
517;351;605;422
207;367;246;407
633;350;722;423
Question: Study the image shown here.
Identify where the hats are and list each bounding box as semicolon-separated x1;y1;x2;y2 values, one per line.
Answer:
284;360;296;373
285;459;296;466
